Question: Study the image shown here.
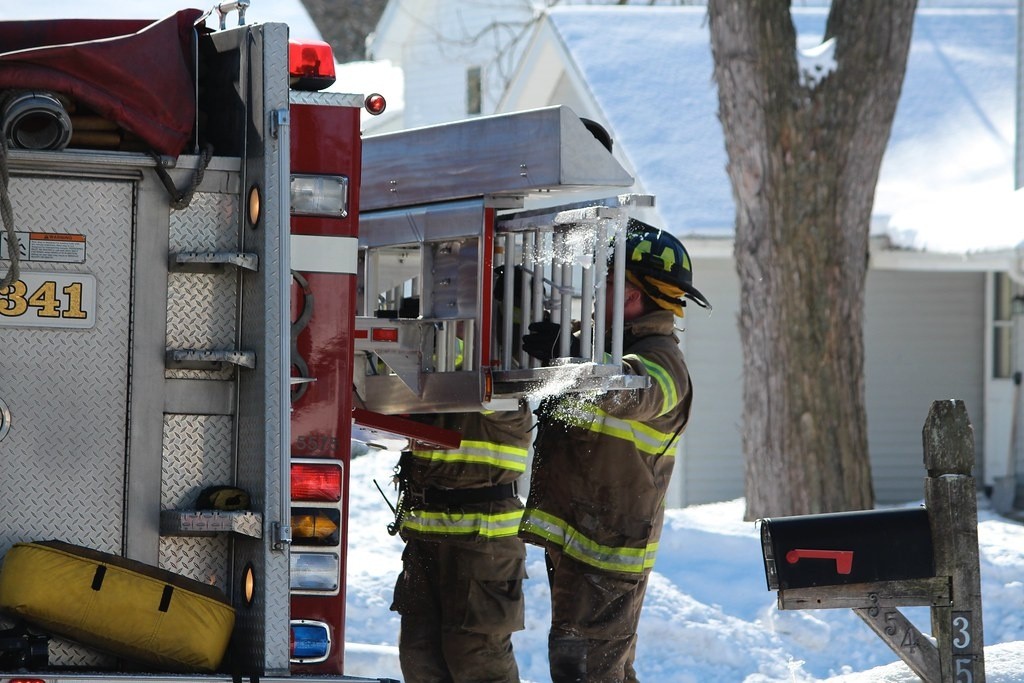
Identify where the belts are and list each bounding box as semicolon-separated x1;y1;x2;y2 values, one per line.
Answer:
407;480;517;506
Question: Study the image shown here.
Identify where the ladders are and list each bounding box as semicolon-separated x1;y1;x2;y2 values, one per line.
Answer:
384;193;655;394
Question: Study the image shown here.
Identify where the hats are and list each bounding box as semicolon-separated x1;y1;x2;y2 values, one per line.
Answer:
625;269;687;318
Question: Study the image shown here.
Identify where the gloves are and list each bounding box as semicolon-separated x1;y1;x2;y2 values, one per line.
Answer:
493;265;546;309
522;311;580;367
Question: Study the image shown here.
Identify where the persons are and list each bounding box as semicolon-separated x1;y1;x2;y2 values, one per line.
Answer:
388;237;547;683
517;219;711;683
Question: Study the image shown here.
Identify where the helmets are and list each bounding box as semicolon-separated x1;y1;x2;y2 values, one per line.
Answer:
593;218;712;309
